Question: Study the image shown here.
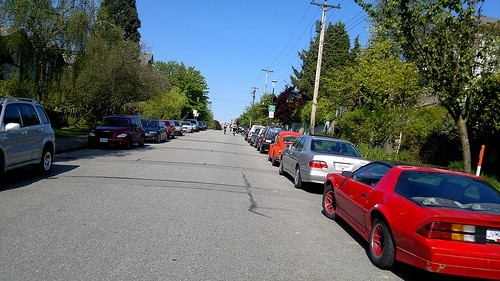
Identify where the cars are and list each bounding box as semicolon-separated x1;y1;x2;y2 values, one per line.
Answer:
162;120;176;141
321;158;500;281
244;124;265;146
279;134;371;189
199;121;206;130
87;115;144;151
0;96;55;180
257;127;281;154
172;120;185;136
187;119;199;131
141;119;167;144
180;121;192;133
268;130;304;167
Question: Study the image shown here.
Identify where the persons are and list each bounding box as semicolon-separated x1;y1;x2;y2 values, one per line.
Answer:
223;122;227;134
229;122;237;134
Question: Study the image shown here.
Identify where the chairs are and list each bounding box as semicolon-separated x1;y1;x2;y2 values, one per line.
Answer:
284;137;289;142
331;146;340;152
439;182;463;201
396;176;410;195
